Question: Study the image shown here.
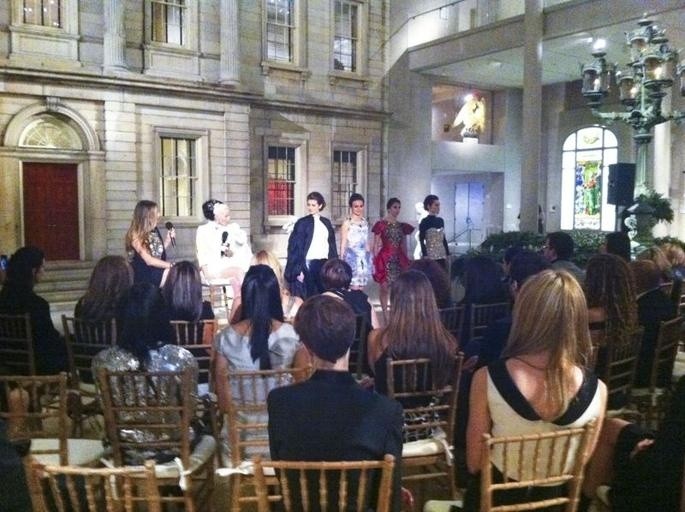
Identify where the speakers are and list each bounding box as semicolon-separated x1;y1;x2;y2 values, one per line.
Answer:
607;163;636;205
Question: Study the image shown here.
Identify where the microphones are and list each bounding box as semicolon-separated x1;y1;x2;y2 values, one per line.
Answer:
220;232;228;257
166;221;177;249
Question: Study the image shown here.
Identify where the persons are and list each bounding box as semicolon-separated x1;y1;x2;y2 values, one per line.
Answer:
581;371;685;511
372;198;415;326
338;194;375;295
211;265;312;443
462;256;515;359
510;253;544;296
409;255;454;333
229;251;304;326
0;245;68;376
502;248;524;281
71;257;135;354
320;258;374;361
543;230;587;296
583;254;638;411
642;246;682;313
126;198;178;288
286;191;339;295
465;267;611;512
657;243;685;309
267;294;404;512
600;232;634;262
196;201;253;296
420;195;451;270
630;260;678;387
158;260;219;385
90;283;224;466
375;271;459;441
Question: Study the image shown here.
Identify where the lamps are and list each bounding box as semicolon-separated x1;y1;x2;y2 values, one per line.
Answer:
576;7;685;260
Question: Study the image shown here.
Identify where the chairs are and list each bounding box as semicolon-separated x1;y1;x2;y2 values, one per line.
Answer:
385;351;464;507
60;310;118;429
480;423;598;512
650;313;682;396
173;318;218;395
604;326;646;425
96;367;192;471
0;373;68;469
20;456;162;512
439;303;465;346
349;314;367;380
0;314;68;429
221;367;314;512
473;303;511;342
251;455;396;512
